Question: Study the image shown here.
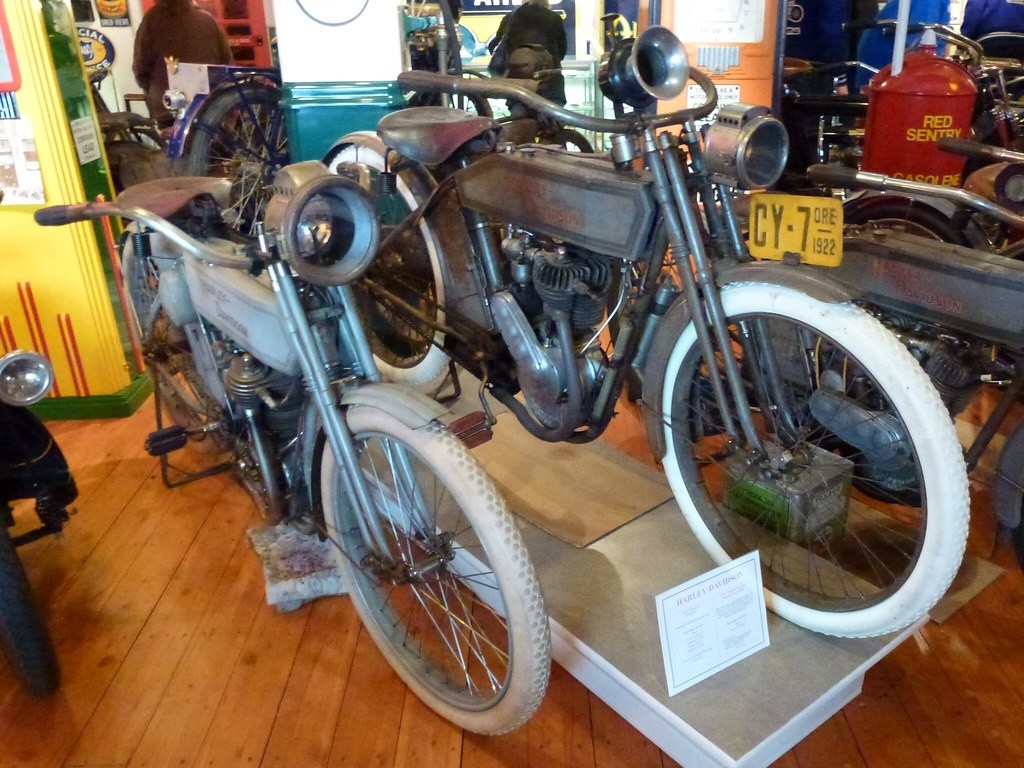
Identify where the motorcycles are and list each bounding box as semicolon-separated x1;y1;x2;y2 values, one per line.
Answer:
313;6;972;643
688;13;1024;554
158;58;284;243
33;163;554;737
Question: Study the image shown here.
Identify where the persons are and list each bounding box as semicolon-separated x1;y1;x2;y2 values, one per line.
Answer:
855;0;951;89
132;0;232;129
959;0;1024;58
490;0;568;114
779;0;847;174
410;0;463;104
